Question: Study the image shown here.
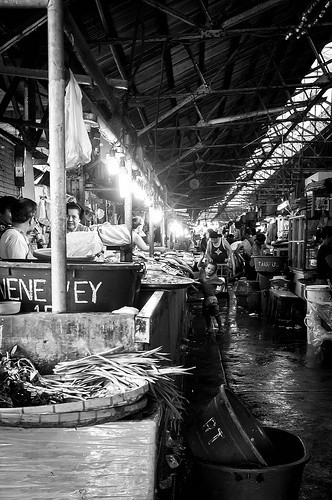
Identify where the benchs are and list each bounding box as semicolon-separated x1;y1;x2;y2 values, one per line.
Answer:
268;287;299;321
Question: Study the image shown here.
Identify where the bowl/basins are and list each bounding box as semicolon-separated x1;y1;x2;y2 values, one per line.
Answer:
198;385;272;467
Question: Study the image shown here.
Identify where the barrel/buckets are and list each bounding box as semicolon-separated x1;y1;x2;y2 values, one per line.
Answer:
305;284;329;346
185;426;311;500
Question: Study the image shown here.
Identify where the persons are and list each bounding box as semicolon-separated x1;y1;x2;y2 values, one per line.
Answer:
197;252;226;337
315;226;332;284
0;196;267;295
